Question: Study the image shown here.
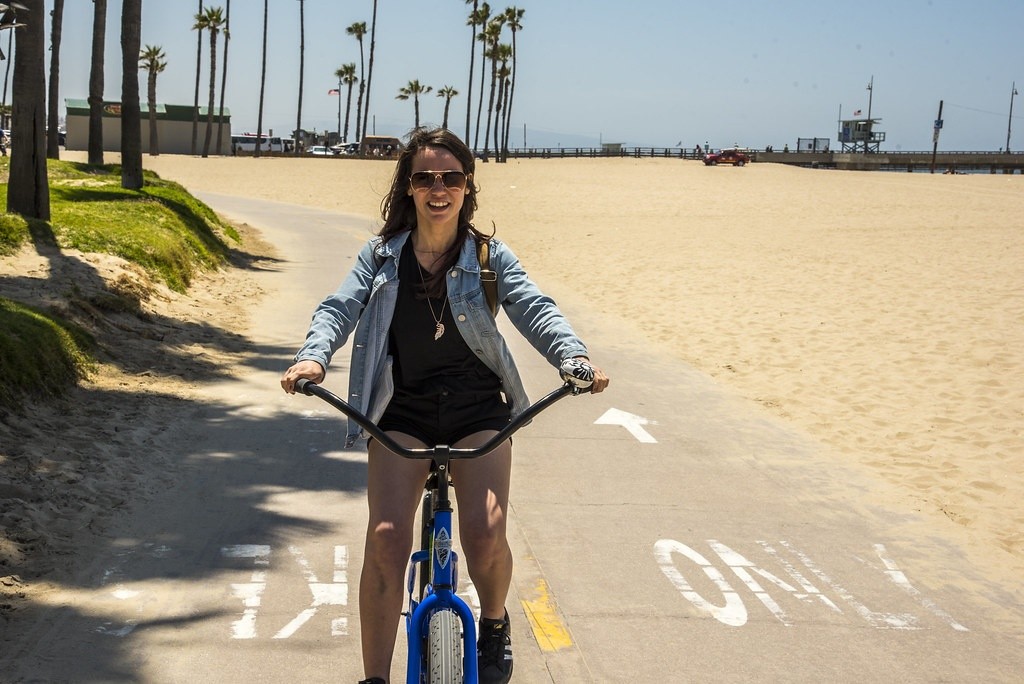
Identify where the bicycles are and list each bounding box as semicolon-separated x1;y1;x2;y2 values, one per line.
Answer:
302;356;594;683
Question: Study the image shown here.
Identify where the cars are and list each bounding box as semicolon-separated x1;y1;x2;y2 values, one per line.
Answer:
330;141;362;156
702;150;750;166
306;144;334;155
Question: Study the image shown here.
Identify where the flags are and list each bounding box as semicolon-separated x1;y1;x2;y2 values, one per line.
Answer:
854;110;862;115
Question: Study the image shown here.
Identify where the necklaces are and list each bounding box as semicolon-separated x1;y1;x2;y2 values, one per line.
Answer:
415;256;448;341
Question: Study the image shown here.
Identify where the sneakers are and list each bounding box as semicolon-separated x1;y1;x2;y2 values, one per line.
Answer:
477;605;513;683
358;678;385;684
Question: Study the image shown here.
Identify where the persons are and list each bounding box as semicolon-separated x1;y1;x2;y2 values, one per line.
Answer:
231;140;237;156
999;147;1010;155
694;142;830;160
280;124;610;684
284;137;393;160
0;128;7;156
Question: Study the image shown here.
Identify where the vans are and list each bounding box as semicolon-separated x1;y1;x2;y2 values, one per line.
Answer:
269;137;296;154
231;135;272;153
364;137;407;155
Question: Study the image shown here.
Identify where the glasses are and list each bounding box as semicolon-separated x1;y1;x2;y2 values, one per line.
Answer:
408;171;467;193
